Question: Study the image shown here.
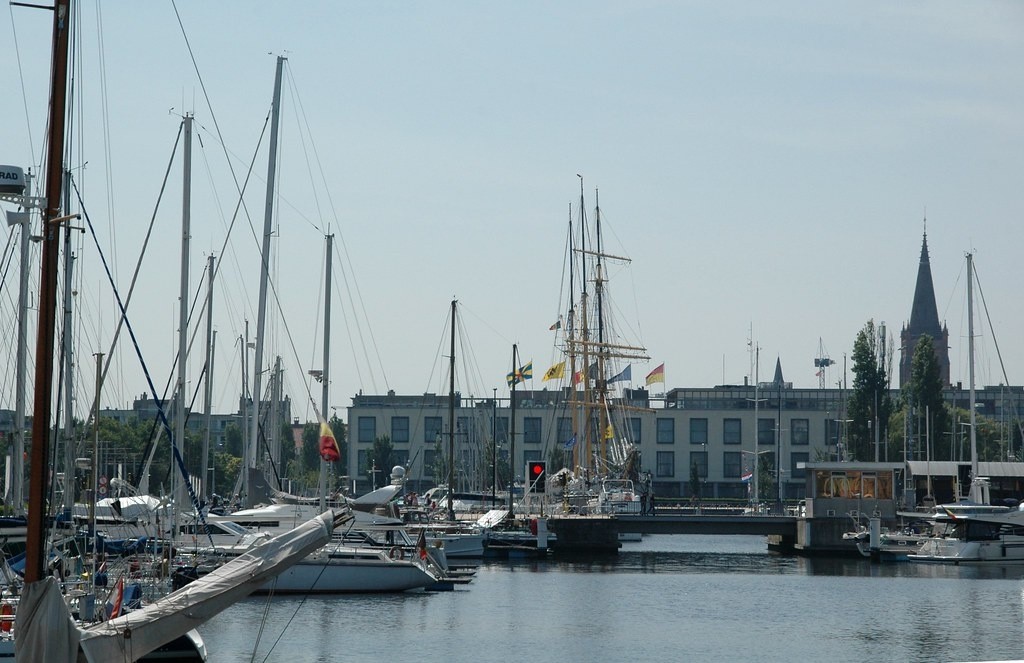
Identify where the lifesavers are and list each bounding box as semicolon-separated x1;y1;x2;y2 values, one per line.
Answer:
389;546;405;560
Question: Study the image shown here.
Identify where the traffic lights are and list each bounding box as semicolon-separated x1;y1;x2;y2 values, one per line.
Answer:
527;460;548;494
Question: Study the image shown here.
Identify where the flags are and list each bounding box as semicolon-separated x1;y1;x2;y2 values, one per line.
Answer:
645;364;663;387
572;362;598;385
563;432;578;448
599;424;614;442
945;509;957;521
742;472;753;483
104;577;124;620
815;370;824;376
315;409;341;462
543;361;564;381
418;528;427;560
506;361;532;389
607;364;631;384
549;320;561;330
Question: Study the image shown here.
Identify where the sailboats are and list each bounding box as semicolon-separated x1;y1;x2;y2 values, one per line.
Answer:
842;239;1024;566
0;0;667;663
739;340;808;518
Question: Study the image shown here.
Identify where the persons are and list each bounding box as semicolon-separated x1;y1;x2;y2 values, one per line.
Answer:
232;494;241;511
210;493;219;511
422;493;431;517
410;493;422;527
402;495;412;525
